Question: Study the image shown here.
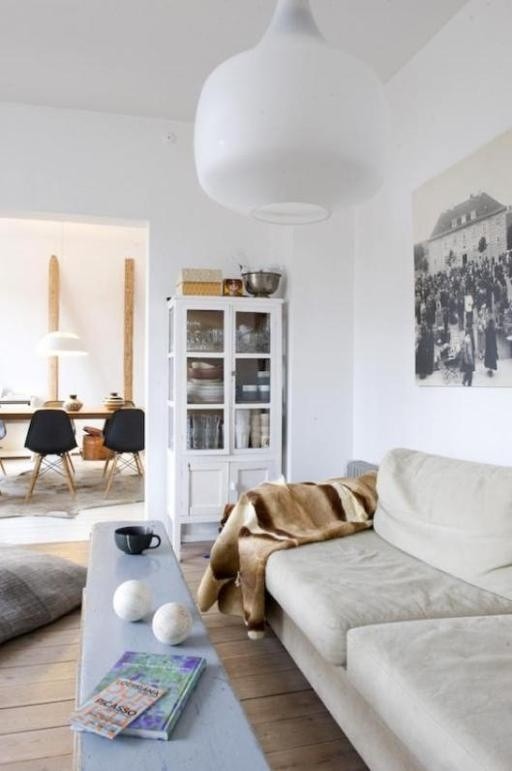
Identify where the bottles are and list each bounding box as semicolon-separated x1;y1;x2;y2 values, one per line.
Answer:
190;415;220;451
243;372;270;402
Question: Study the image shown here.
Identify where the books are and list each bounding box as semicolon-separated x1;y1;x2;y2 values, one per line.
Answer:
67;676;168;741
76;649;208;743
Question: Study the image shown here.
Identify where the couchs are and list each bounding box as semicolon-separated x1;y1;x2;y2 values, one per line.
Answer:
260;450;512;771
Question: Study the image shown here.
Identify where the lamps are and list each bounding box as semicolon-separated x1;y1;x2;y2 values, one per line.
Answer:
37;333;90;358
195;0;386;226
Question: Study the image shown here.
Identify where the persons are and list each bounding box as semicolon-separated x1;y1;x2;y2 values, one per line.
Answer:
415;248;512;387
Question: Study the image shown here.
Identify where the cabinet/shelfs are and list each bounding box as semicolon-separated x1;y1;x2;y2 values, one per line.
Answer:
166;295;286;563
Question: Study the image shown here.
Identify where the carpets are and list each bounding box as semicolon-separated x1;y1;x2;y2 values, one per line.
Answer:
0;467;146;520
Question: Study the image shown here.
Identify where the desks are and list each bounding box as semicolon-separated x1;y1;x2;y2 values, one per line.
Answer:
0;405;118;419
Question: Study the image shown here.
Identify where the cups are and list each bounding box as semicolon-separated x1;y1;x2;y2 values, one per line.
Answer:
115;525;162;557
234;409;270;449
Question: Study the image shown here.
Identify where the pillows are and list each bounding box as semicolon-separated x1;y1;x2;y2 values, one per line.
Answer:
0;555;87;643
373;449;512;600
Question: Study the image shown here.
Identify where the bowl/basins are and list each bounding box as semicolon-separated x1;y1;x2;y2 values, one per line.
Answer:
189;367;222;403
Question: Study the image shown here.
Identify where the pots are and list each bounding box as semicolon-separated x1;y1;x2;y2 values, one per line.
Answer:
242;271;281;297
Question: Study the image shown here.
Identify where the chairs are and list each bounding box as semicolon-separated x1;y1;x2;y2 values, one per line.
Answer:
103;401;145;501
24;400;79;499
0;418;7;475
